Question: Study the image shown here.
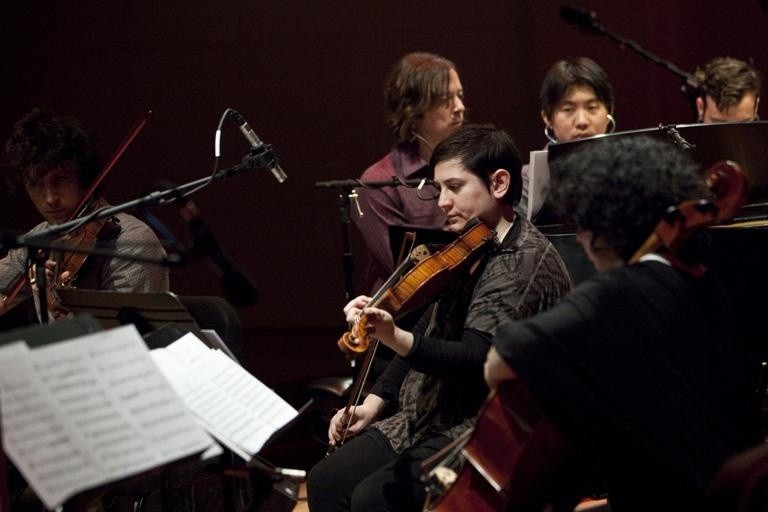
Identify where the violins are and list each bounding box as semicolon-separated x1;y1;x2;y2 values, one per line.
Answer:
48;197;122;328
337;222;499;355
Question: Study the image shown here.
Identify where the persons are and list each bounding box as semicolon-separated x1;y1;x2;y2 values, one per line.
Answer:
305;125;573;512
484;135;768;512
694;57;762;125
349;52;465;298
0;106;169;511
518;56;613;214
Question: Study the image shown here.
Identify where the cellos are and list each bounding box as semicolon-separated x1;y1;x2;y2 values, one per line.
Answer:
428;160;751;512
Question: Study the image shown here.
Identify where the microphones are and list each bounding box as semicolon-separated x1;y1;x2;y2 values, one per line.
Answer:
557;4;601;32
175;195;258;308
230;111;288;183
